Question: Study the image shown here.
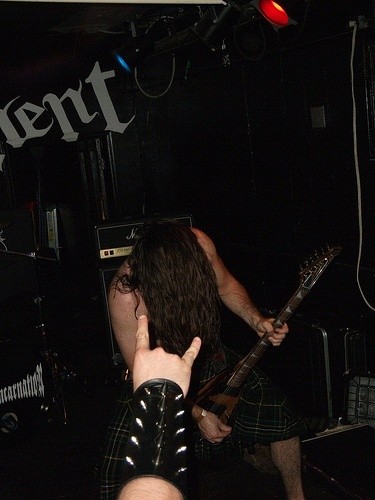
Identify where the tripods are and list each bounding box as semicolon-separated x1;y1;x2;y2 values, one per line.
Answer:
1;147;96;426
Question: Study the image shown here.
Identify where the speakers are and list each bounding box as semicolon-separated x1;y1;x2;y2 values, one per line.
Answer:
85;256;131;387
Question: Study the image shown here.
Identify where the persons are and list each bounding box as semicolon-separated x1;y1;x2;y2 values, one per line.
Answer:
117;314;202;500
94;219;306;500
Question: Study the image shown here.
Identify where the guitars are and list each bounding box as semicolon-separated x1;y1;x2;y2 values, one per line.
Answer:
190;243;342;446
